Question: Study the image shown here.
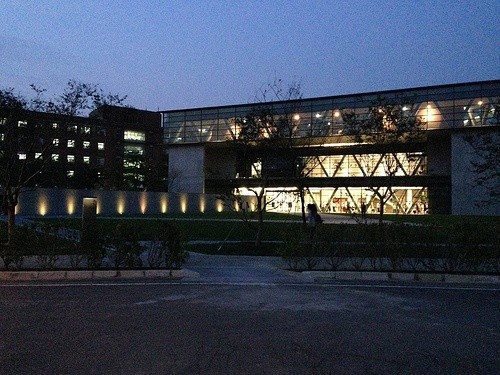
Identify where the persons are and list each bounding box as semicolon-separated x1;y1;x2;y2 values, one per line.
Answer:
306;204;323;241
277;201;428;218
